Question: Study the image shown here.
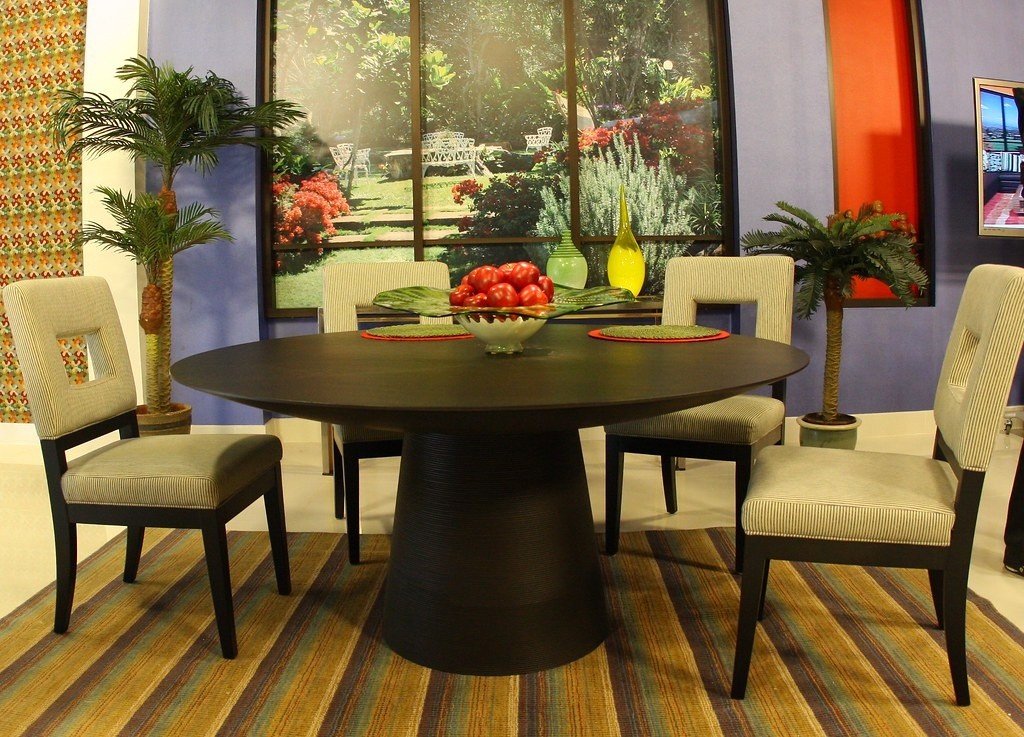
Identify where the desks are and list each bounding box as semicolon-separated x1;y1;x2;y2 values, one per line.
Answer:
167;317;808;676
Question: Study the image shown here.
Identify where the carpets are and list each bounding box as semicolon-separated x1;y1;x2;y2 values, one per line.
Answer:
0;522;1022;736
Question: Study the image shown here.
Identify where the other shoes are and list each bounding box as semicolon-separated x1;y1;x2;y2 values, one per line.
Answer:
1005;564;1024;574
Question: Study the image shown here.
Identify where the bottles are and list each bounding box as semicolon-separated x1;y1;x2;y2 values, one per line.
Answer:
607;184;645;298
546;229;588;290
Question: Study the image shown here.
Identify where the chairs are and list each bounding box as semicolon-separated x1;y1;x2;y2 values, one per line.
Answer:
323;261;452;564
2;273;294;661
607;253;797;574
730;263;1023;707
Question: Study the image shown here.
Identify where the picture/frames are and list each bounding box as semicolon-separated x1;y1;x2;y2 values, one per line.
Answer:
971;77;1024;238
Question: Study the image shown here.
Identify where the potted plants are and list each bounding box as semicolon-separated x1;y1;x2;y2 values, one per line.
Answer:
742;204;927;448
47;53;305;438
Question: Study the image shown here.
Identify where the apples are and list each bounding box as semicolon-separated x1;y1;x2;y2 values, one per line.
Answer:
450;260;553;322
